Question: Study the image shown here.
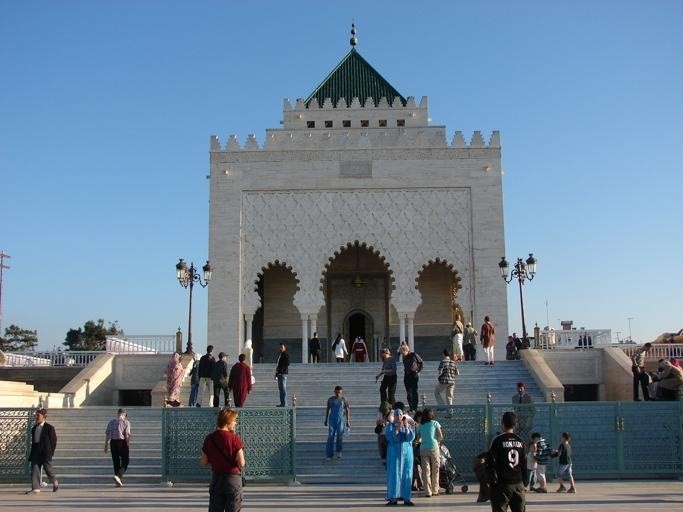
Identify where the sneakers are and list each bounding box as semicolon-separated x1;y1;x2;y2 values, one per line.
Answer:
567;487;576;493
53;480;58;492
26;489;41;495
337;451;342;458
112;475;123;487
534;486;547;493
556;485;566;492
485;362;490;365
327;457;334;461
490;362;494;365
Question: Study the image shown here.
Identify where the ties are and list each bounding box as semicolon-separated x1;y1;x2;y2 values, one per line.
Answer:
519;395;522;404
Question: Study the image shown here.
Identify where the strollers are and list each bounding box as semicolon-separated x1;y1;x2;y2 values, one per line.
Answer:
415;442;469;494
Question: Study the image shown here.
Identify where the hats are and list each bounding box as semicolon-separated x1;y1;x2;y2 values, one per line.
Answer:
517;382;526;388
219;352;229;358
36;409;47;418
118;408;128;417
394;408;403;415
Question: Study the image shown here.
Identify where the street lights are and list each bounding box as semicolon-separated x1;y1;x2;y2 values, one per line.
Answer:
176;259;212;354
498;253;536;348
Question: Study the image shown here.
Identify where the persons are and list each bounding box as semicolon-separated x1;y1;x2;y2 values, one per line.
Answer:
506;333;530;360
352;337;366;362
451;314;464;361
399;343;423;412
189;353;200;405
376;348;396;404
198;345;217;407
310;332;321;363
435;349;459;418
463;322;478;361
324;386;350;461
228;354;253;407
331;333;349;362
201;408;246;511
105;409;131;488
25;408;58;494
512;383;537;437
648;358;683;400
275;343;290;407
164;353;186;407
631;343;657;401
480;316;494;365
211;352;232;407
374;401;581;503
578;335;592;348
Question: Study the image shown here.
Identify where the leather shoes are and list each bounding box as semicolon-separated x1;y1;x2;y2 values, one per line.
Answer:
276;403;287;407
432;494;441;496
404;500;414;506
634;398;642;402
644;397;657;401
426;494;431;497
386;500;397;506
